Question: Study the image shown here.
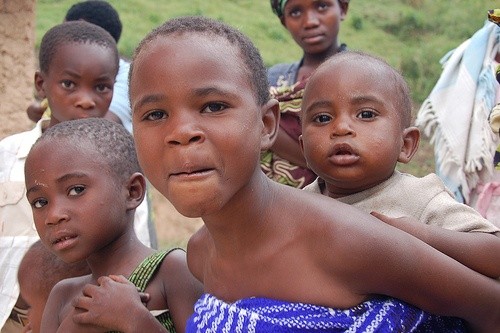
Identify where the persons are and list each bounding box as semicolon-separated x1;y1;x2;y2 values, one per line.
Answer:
26;0;131;135
1;18;153;333
261;1;349;191
23;118;203;333
17;240;94;333
129;15;500;333
296;49;500;282
